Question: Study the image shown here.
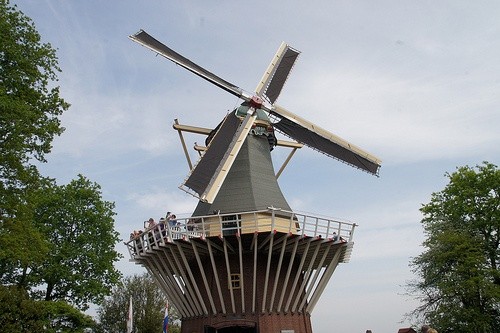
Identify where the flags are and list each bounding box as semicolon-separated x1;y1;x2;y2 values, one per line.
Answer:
127;295;133;332
163;302;169;332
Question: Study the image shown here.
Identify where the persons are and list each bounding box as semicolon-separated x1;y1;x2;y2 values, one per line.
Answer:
129;210;198;254
332;231;343;240
